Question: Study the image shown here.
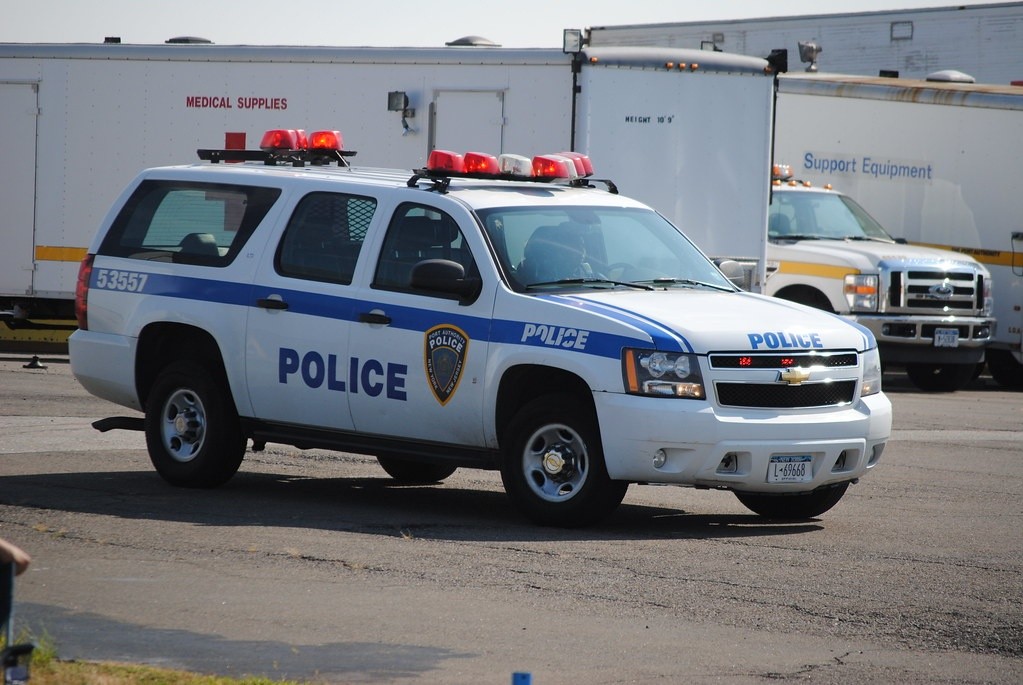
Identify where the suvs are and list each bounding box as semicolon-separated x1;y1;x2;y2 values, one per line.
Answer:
758;163;999;396
66;127;894;528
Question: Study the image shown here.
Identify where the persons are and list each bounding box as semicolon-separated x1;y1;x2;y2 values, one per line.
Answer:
534;222;608;283
0;537;32;639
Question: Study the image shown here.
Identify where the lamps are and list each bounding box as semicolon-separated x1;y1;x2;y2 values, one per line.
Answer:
388;91;413;135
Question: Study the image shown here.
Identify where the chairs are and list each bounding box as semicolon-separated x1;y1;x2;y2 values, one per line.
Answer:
384;216;436;294
